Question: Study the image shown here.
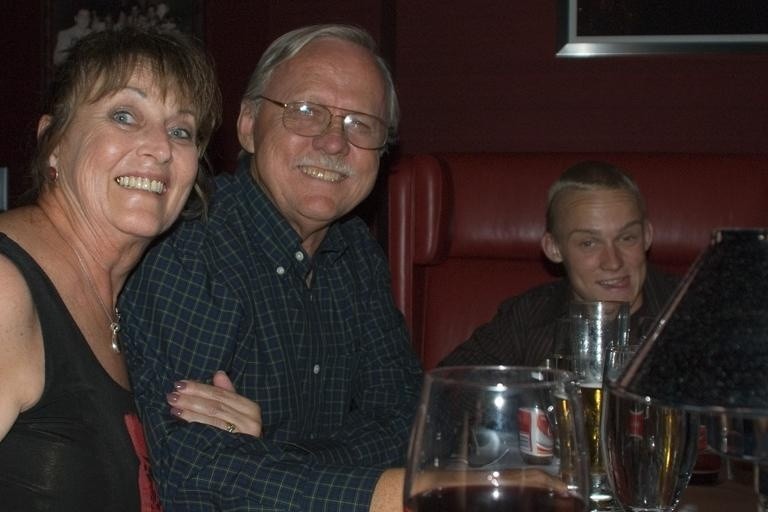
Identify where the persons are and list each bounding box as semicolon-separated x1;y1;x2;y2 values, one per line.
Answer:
0;6;262;512
430;160;679;459
117;26;573;512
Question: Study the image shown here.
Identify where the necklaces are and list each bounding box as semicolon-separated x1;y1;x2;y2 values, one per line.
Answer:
67;243;122;356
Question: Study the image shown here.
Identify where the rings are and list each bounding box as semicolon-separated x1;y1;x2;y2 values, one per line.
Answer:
225;422;234;436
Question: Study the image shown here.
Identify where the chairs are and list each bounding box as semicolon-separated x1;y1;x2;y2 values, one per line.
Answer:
387;152;768;374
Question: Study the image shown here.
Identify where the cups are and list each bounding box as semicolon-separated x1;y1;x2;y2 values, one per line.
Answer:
570;298;631;389
538;346;721;512
400;363;590;511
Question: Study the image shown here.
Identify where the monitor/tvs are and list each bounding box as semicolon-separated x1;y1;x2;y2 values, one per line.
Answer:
556;0;768;58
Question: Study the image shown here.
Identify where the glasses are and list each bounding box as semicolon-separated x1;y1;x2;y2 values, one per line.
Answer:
255;94;389;150
